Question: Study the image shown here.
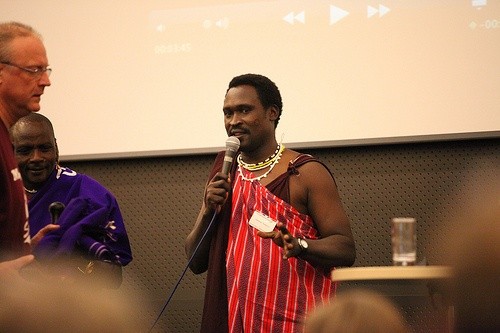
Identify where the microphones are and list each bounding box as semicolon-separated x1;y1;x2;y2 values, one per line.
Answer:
222;136;240;175
48;201;66;224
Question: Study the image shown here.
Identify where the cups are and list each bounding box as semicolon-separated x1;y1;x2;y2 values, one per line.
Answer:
391;218;416;266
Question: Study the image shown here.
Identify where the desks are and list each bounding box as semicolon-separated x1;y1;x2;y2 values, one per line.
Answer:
329;264;458;333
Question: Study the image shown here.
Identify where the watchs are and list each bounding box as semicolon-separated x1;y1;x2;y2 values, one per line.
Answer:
294;237;308;260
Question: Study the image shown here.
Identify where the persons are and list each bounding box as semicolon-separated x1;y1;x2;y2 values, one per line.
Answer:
9;113;133;289
184;74;355;333
0;22;61;294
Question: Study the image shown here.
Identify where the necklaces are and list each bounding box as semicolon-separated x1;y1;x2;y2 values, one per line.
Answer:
23;186;38;194
237;143;284;182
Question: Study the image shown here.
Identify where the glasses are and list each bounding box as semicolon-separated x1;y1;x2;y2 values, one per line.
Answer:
1;57;54;78
